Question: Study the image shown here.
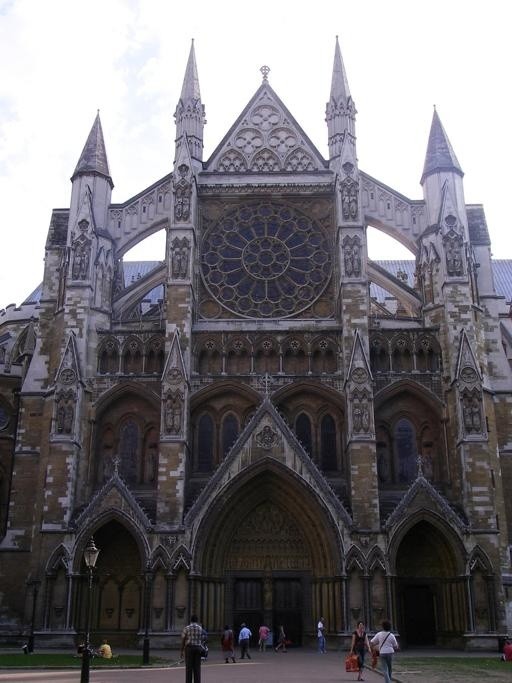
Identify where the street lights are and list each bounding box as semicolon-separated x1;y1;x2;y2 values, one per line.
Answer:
78;534;101;682
27;572;43;652
139;560;156;666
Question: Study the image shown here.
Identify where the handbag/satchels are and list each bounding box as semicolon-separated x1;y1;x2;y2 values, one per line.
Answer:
344;654;360;671
285;639;292;646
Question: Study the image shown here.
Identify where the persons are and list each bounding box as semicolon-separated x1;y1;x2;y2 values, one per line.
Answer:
257;623;271;653
220;624;237;663
179;614;209;682
237;621;253;659
370;620;398;683
274;622;289;653
316;616;328;654
96;638;113;658
344;620;373;681
500;639;512;661
